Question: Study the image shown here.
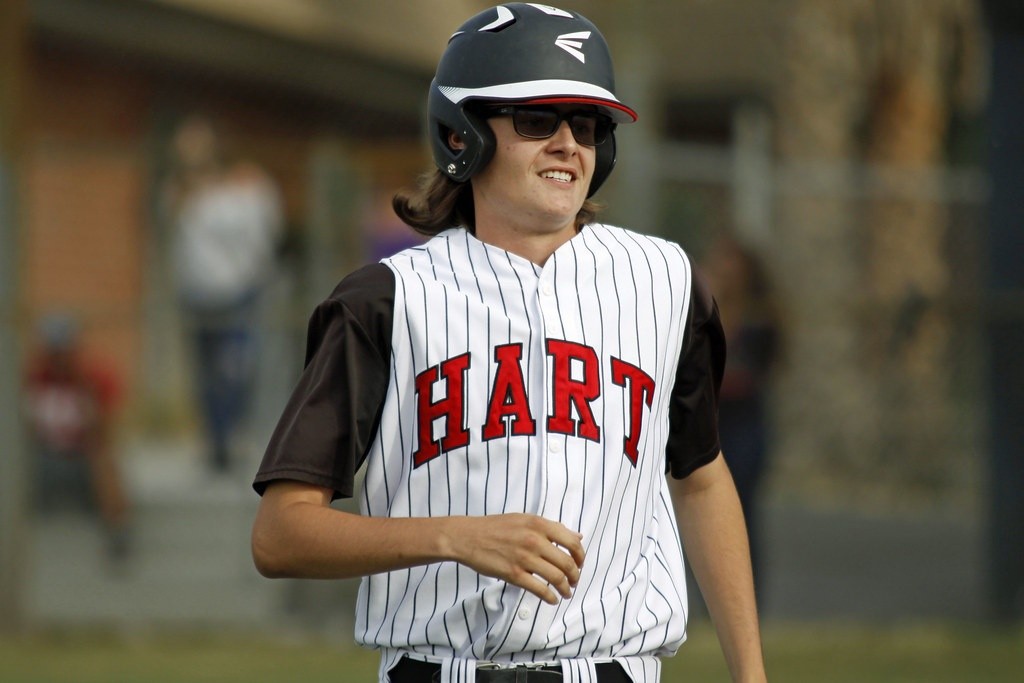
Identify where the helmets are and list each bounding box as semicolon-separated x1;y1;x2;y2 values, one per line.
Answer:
426;2;639;200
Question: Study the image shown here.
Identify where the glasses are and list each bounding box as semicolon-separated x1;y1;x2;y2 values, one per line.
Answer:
482;103;618;146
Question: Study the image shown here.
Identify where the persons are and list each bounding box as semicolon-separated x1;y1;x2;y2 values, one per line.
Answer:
25;313;135;552
254;0;767;683
171;123;279;466
701;242;782;571
365;190;427;258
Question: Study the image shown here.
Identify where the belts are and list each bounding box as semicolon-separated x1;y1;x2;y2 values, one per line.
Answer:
387;655;633;683
